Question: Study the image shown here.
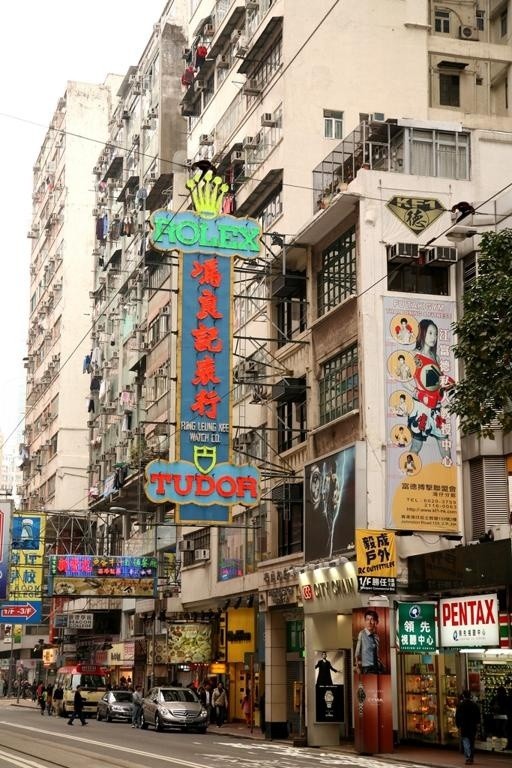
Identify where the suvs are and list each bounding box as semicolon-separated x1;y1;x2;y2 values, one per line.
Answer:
139;687;210;734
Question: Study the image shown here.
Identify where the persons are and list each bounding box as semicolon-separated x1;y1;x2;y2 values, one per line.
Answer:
314;652;343;686
241;688;255;728
490;686;508;751
66;686;88;726
3;678;65;717
113;675;228;728
394;318;454;475
355;612;386;674
457;688;479;764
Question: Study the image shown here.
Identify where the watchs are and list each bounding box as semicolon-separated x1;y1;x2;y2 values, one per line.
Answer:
356;681;367;717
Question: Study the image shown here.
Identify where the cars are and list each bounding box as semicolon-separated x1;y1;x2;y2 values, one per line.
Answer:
96;690;135;723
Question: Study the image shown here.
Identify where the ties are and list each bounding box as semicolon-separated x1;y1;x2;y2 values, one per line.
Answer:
369;634;378;667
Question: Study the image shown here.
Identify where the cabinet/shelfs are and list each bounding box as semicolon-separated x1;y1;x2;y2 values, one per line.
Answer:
400;651;446;745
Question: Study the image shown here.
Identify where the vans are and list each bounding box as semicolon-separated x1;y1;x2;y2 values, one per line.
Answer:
52;661;111;718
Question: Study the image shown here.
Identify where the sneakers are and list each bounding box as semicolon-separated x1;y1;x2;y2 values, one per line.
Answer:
82;722;88;726
68;722;73;725
131;724;140;728
465;756;473;764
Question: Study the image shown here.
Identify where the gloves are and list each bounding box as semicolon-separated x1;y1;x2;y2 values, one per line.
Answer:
406;324;414;334
412;387;439;408
439;375;456;392
395;326;401;334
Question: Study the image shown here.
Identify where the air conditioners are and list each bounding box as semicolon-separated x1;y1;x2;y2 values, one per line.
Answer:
460;23;479;41
180;0;259;92
230;136;257;163
261;113;277;126
245;360;258;373
183;159;194;169
87;80;172;498
199;134;215;145
